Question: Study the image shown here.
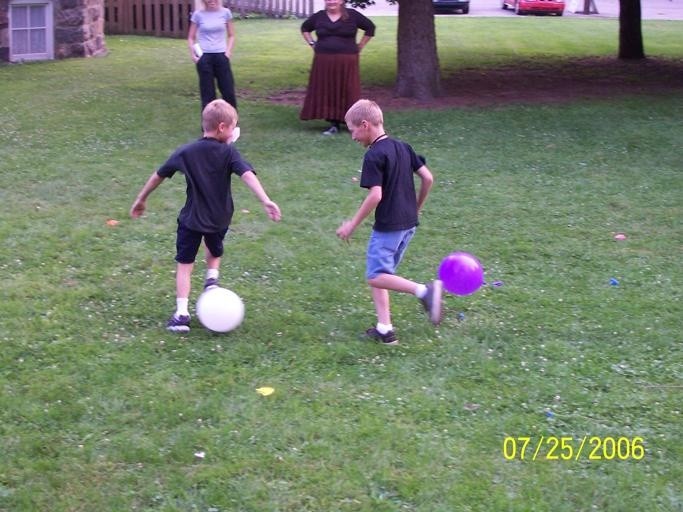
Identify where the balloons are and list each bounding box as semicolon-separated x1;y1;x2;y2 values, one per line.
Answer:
437;255;483;297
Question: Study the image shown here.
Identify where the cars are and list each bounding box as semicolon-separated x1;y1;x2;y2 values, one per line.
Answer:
432;0;469;13
502;0;565;16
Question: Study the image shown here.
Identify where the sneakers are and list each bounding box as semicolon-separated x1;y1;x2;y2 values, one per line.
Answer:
164;314;192;332
323;125;340;135
232;127;241;142
365;322;399;347
417;279;442;325
204;277;218;290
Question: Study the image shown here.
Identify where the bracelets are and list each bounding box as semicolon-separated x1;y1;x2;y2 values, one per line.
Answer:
308;41;315;46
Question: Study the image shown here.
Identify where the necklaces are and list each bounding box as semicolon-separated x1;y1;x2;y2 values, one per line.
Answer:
370;132;386;145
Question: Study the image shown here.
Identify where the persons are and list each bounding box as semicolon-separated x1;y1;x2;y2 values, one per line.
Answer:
131;98;282;333
335;99;443;347
186;1;238;132
299;0;376;136
